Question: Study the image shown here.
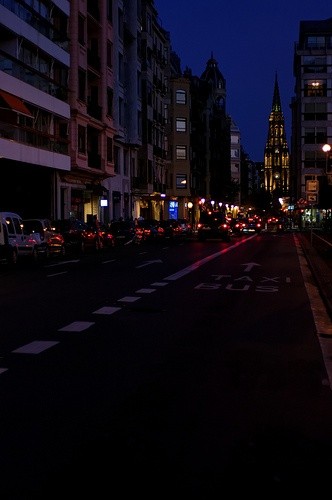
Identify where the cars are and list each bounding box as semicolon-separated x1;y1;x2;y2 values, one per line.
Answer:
0;210;286;262
